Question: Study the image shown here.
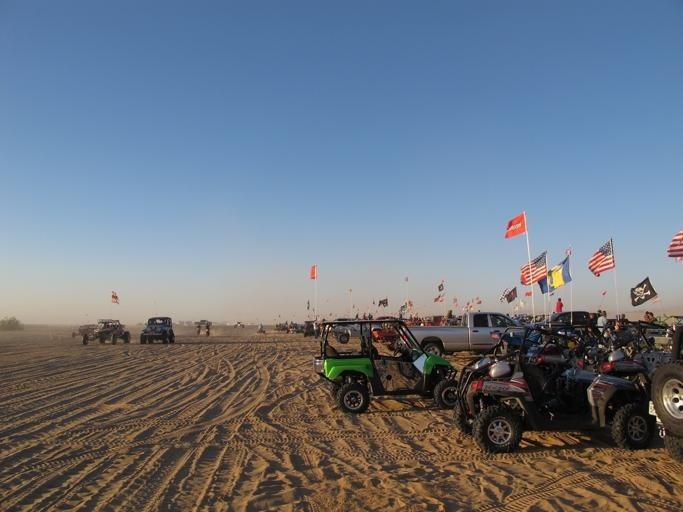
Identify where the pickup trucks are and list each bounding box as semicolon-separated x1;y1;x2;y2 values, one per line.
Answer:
399;310;539;364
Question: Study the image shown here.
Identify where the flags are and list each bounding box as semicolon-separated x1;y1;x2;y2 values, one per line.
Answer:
589;238;615;277
667;231;683;261
310;265;317;280
111;291;120;304
505;213;525;238
539;255;571;294
630;276;657;306
521;251;546;285
378;276;532;315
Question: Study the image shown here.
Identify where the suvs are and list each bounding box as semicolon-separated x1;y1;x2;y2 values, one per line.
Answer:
636;319;683;463
82;319;131;346
140;317;176;344
332;318;373;344
312;316;467;415
452;326;658;456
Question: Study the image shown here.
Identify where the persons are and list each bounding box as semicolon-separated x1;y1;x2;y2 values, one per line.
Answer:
644;312;657;323
597;310;608;332
556;298;563;312
